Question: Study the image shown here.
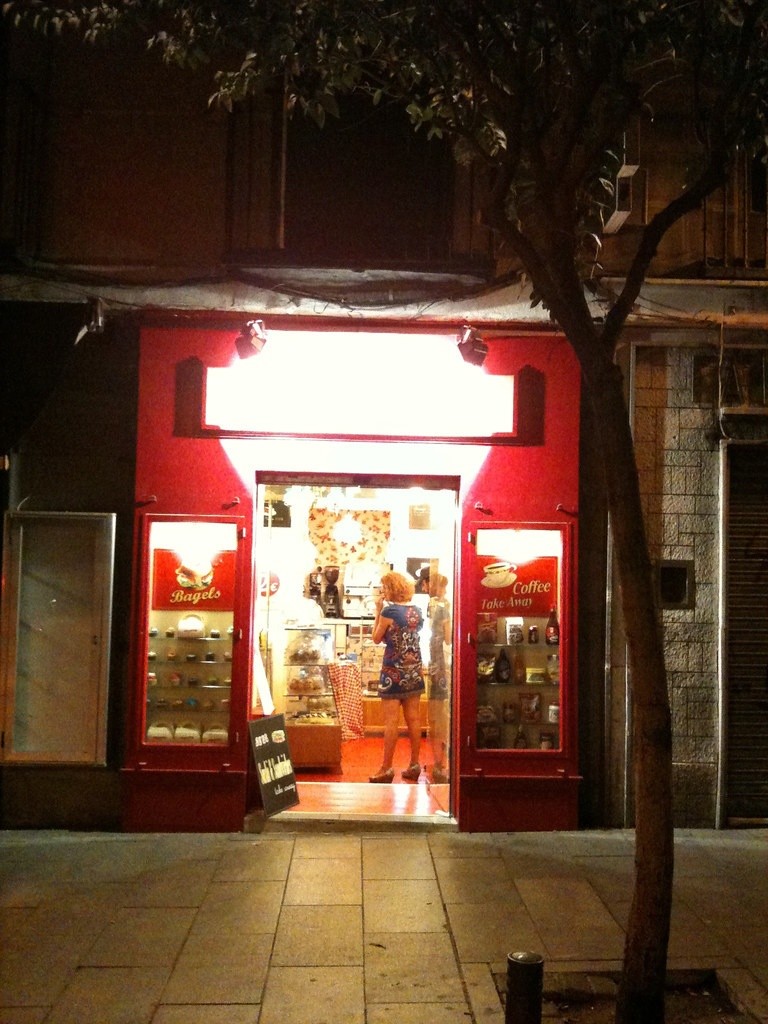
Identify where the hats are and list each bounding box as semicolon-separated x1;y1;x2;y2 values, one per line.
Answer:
417;567;429;585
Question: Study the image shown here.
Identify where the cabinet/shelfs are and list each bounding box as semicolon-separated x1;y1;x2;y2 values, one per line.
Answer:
283;625;343;775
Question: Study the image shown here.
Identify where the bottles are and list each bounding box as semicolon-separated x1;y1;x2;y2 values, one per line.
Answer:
513;725;527;749
529;626;539;644
496;648;511;683
545;609;559;646
512;647;525;682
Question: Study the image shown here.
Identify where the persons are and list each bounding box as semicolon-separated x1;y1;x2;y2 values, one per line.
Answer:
427;574;452;783
368;572;426;783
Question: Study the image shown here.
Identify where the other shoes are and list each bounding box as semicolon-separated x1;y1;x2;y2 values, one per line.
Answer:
432;766;448;784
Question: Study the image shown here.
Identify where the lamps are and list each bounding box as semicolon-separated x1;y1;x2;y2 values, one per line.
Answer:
455;325;489;366
235;319;268;359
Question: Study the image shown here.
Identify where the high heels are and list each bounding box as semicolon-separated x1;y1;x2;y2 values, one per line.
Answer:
369;767;394;783
401;763;421;782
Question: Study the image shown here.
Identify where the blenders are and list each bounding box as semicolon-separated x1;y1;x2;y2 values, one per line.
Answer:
324;566;340;617
310;573;322;605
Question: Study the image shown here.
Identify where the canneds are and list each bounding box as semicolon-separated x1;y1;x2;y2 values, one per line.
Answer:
528;625;539;643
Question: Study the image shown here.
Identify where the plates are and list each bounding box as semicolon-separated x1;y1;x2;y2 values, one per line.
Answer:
480;572;517;588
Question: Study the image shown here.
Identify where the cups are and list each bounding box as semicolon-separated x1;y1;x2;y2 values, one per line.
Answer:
549;702;559;723
483;562;517;582
